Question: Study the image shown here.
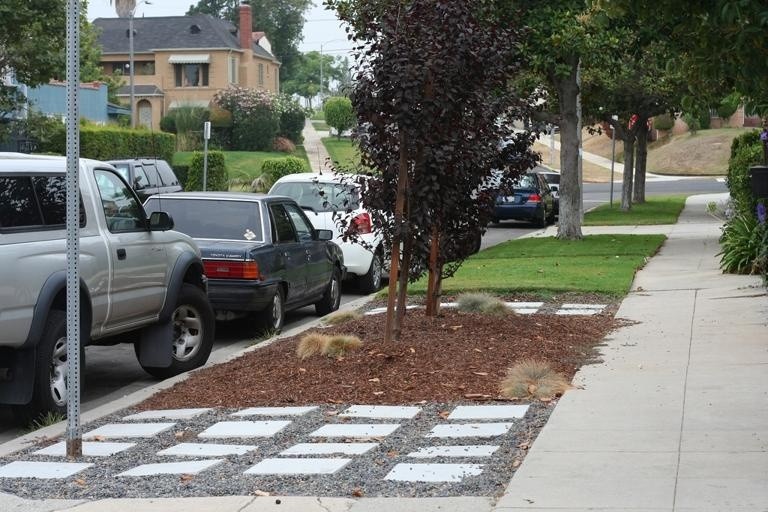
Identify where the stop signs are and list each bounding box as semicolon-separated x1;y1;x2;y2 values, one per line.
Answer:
628;115;651;140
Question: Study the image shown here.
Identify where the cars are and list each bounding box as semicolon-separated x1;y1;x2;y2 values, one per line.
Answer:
478;167;557;229
537;170;561;217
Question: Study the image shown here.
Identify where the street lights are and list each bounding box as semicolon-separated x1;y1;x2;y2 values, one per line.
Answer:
128;1;157;129
608;110;618;204
316;38;351;112
